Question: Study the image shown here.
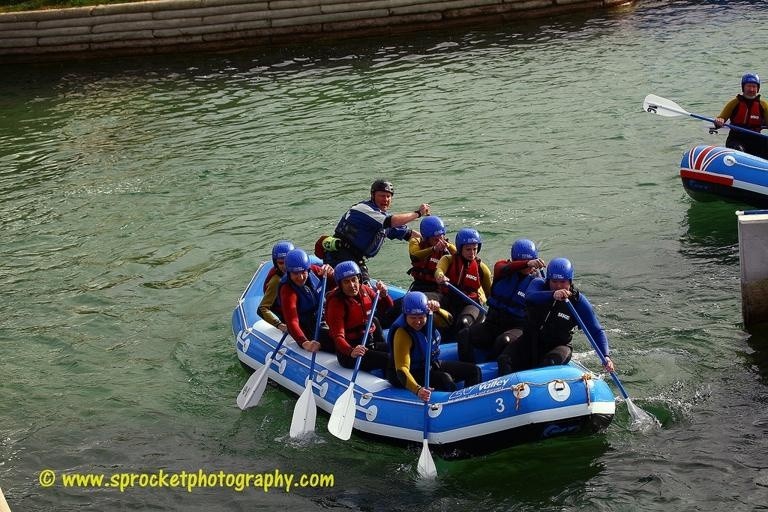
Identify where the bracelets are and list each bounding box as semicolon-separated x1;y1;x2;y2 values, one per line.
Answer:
413;210;421;217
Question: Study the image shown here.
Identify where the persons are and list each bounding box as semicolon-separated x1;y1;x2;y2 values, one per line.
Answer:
713;72;768;158
325;260;394;378
255;240;295;336
408;215;455;303
384;291;480;403
445;238;545;365
323;179;432;283
497;257;614;376
277;248;338;355
432;228;492;344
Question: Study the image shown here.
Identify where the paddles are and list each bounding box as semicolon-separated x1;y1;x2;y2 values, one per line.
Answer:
561;297;656;438
417;312;438;491
236;332;288;411
642;93;767;139
290;272;329;439
327;290;380;440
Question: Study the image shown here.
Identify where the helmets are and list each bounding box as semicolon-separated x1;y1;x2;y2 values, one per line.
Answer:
370;178;394;197
284;248;312;275
741;71;761;94
511;239;539;260
333;260;364;286
420;215;447;239
401;290;430;316
455;227;482;254
271;240;295;269
546;256;573;286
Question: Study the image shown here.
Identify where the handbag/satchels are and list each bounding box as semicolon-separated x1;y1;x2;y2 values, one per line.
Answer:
313;233;345;259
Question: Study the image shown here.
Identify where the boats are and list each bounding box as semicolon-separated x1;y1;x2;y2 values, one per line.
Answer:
231;254;617;460
679;144;768;210
